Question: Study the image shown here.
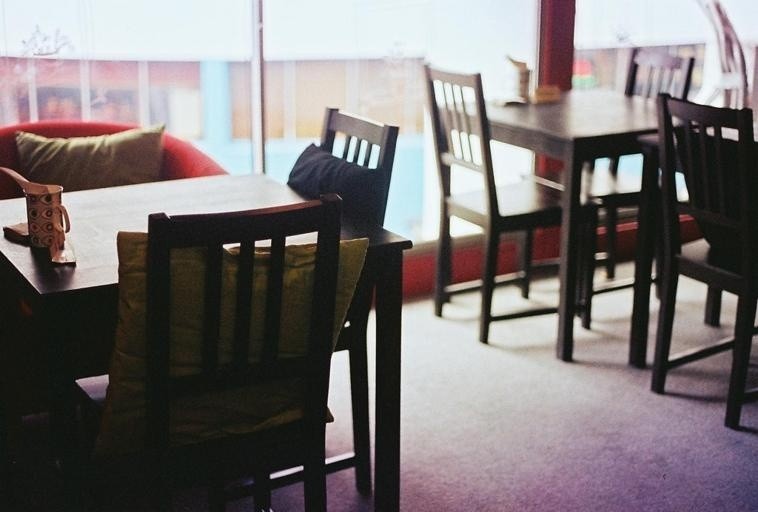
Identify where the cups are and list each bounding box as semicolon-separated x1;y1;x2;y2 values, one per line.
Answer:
25;185;69;251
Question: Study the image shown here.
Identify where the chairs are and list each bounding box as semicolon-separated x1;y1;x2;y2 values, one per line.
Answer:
47;192;343;512
577;49;699;330
423;65;594;344
244;108;398;503
648;89;758;428
0;121;231;199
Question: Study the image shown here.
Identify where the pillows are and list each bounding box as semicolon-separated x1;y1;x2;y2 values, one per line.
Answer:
13;126;169;192
285;142;383;217
100;232;370;449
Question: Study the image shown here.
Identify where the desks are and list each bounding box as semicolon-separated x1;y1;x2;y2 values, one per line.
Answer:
440;86;692;367
629;125;756;369
1;173;411;511
